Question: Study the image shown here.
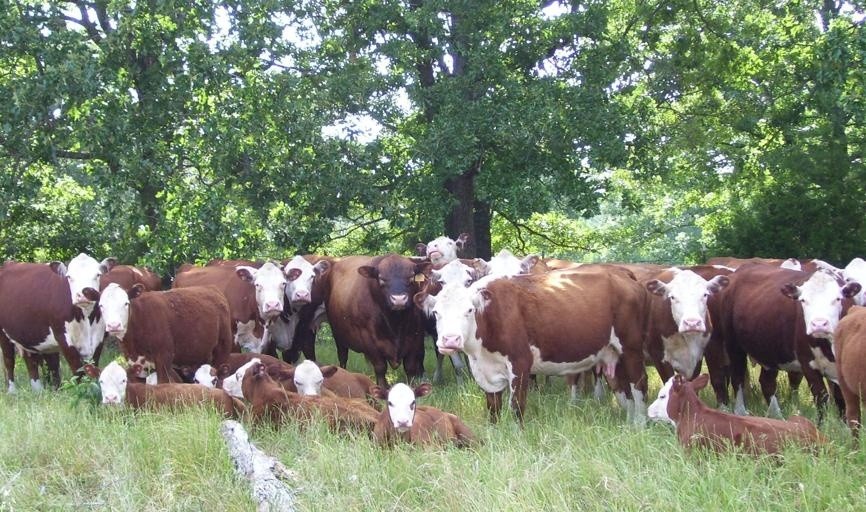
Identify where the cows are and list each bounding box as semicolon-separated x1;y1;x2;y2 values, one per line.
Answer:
0;231;866;459
